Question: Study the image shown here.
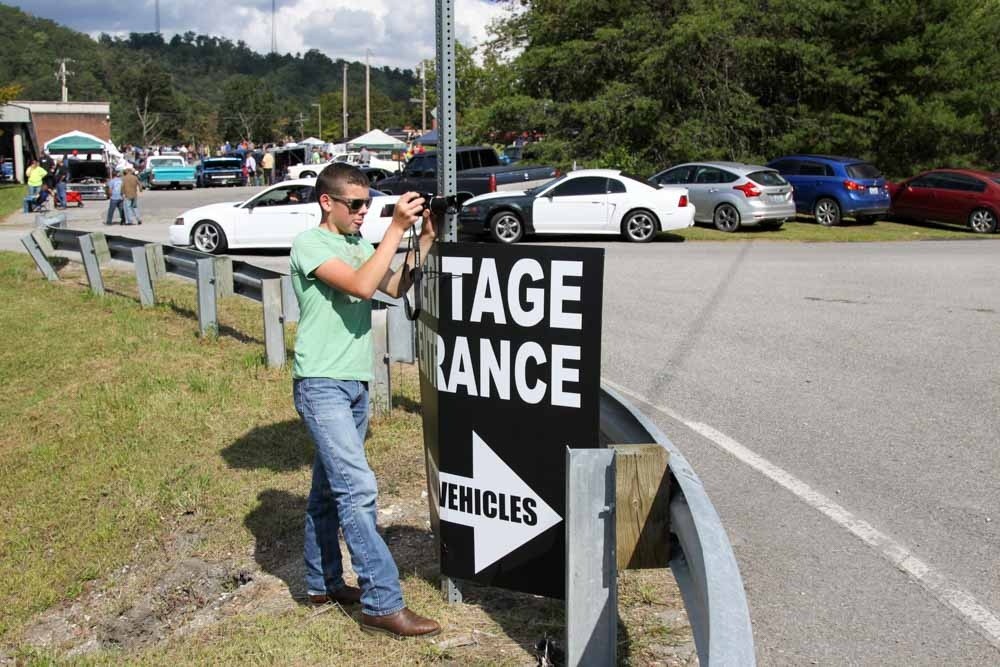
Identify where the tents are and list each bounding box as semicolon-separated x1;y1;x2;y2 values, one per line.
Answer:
346;128;407;170
44;130;112;179
413;129;438;146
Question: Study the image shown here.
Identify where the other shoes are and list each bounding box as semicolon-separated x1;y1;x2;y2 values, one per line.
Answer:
102;221;112;225
120;221;125;225
138;221;142;224
126;223;135;225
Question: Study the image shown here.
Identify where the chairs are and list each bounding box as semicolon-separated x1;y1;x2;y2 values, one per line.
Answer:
29;193;49;213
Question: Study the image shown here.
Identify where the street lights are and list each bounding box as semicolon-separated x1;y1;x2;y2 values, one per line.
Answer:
311;104;322;140
410;97;427;137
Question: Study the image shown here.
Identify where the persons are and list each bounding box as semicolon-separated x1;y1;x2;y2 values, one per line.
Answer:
410;142;424;155
310;146;320;164
118;139;274;186
23;160;68;212
288;163;443;639
277;135;295;147
359;146;370;167
102;168;142;226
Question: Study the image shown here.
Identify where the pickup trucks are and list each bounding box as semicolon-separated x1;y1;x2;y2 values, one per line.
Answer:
370;145;561;212
384;127;408;142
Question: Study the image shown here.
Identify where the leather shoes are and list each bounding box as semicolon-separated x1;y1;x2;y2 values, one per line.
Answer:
362;608;442;639
308;585;362;605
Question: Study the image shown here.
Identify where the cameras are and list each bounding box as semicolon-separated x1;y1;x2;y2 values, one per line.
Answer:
408;192;460;216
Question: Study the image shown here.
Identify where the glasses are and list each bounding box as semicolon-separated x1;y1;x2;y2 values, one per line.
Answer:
321;192;373;212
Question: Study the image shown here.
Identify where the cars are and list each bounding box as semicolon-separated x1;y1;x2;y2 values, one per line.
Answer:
0;157;17;183
456;169;696;243
194;156;244;189
757;153;892;227
871;168;1000;234
497;134;541;165
168;176;423;256
645;160;797;234
140;155;196;191
287;152;406;186
